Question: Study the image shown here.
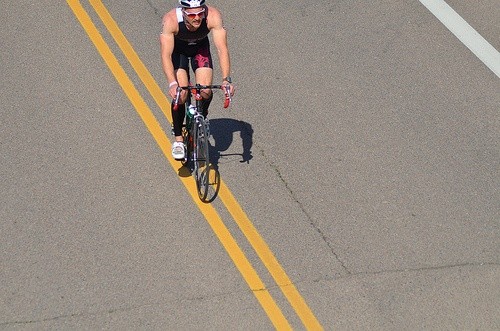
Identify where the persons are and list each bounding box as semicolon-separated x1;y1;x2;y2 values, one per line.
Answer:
160;0;235;159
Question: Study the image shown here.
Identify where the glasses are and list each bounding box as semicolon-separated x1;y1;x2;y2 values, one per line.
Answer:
184;8;205;18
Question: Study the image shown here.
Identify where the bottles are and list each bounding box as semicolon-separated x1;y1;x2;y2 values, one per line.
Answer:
185;104;197;131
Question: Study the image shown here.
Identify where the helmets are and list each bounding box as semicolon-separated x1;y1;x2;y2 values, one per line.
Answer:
178;0;205;8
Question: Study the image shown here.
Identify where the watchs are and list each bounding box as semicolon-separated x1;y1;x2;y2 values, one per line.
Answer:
222;77;231;83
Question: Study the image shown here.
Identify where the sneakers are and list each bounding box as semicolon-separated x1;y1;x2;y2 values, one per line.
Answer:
172;142;185;159
199;117;210;137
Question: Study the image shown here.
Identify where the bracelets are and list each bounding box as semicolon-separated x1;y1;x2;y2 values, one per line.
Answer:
169;81;177;88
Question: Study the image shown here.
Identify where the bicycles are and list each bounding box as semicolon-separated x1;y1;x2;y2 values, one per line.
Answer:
174;83;232;203
